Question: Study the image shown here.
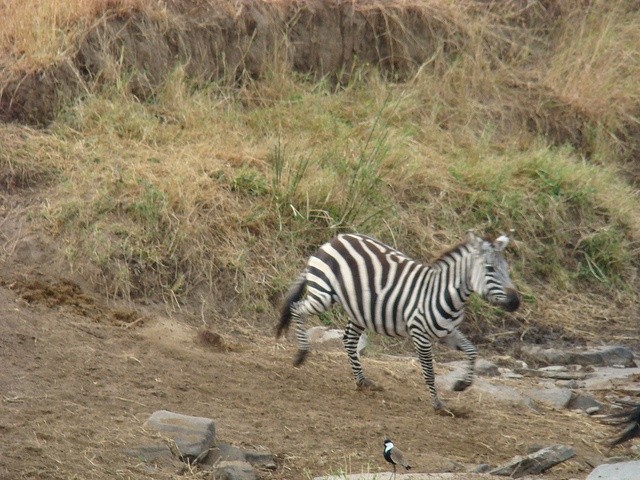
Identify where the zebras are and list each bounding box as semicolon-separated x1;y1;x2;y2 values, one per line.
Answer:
272;227;524;420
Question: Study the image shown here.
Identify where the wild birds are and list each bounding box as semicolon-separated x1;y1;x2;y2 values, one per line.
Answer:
383;439;413;480
